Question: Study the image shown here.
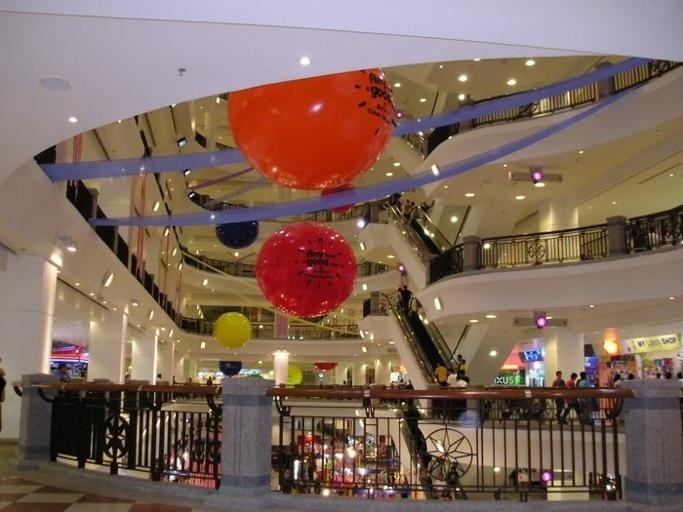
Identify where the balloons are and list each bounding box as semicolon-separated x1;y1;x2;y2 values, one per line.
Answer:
284;364;302;387
314;362;337;370
218;361;243;376
225;66;394;192
320;183;358;214
212;310;252;349
255;220;358;318
215;216;259;248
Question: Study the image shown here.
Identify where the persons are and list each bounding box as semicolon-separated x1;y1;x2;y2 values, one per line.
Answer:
393;194;435;311
206;375;213;387
53;359;71;382
125;374;131;382
397;352;683;426
427;457;528;502
579;220;683;261
156;373;162;384
